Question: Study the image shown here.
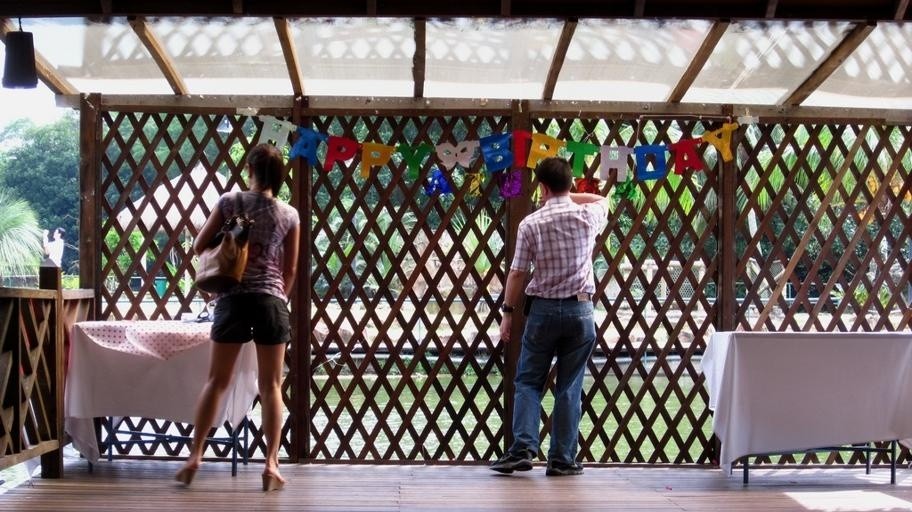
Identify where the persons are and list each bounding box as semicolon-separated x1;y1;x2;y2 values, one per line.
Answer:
173;142;302;492
489;156;612;475
43;227;66;269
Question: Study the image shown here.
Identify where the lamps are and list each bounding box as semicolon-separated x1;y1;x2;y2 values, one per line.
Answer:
1;15;38;89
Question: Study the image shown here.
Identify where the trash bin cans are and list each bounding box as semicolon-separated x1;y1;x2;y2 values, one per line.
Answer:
154;277;168;297
130;277;141;291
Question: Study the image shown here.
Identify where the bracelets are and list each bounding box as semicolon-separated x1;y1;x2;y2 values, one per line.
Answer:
500;302;516;313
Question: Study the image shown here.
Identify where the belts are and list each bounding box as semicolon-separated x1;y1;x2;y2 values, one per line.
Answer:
530;292;592;304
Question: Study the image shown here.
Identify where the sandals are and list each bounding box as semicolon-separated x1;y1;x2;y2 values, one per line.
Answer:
262;469;285;492
172;459;200;487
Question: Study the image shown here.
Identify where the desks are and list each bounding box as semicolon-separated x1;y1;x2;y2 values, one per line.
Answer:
63;320;261;476
700;331;912;483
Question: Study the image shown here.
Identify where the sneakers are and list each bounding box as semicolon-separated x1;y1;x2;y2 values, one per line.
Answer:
488;445;535;474
544;453;585;477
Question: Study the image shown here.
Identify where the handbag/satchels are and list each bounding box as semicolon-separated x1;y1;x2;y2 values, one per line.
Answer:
191;190;256;295
